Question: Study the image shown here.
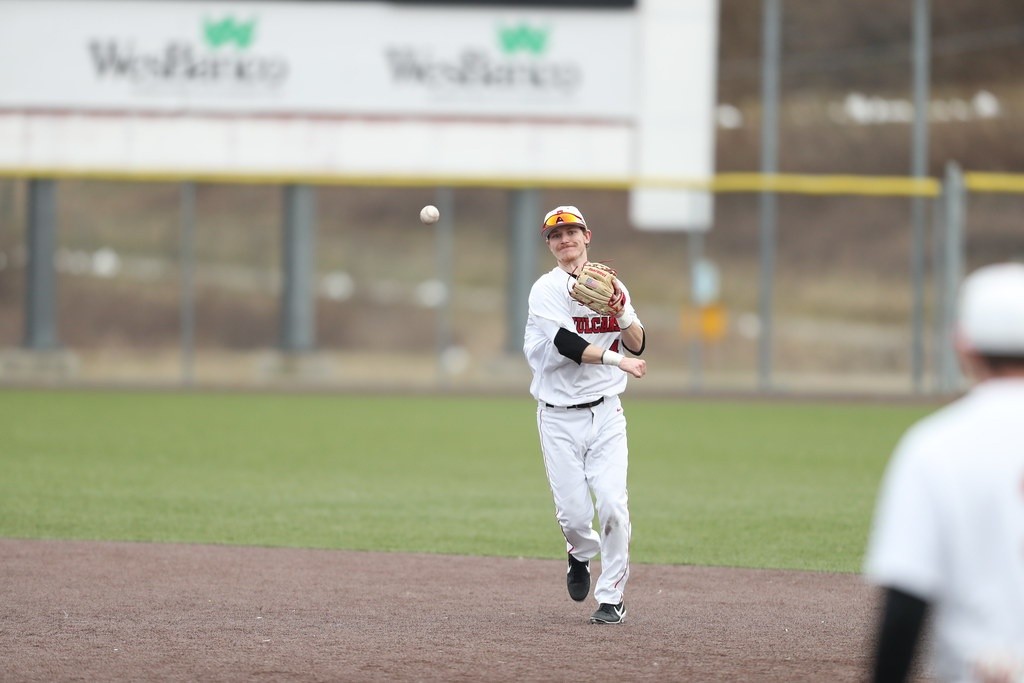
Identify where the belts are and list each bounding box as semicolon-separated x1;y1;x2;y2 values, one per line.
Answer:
546;396;604;409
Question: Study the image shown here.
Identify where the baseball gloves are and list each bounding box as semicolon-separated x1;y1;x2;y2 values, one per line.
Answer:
566;260;626;317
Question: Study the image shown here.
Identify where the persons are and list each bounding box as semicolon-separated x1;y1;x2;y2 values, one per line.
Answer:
860;262;1022;683
519;205;647;627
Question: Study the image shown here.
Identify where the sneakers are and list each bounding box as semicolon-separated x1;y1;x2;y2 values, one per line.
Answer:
589;601;626;624
566;553;592;602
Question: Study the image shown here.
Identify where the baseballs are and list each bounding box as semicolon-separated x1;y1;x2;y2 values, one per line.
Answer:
420;203;441;224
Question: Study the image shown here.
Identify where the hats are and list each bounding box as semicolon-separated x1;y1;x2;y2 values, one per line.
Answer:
952;262;1024;357
541;205;587;241
